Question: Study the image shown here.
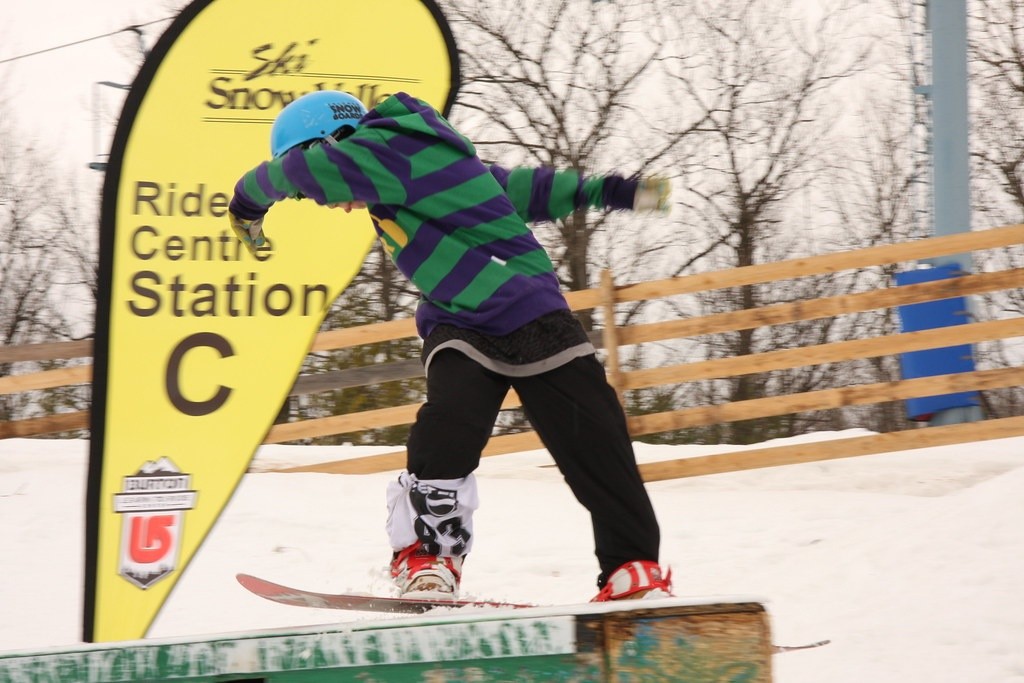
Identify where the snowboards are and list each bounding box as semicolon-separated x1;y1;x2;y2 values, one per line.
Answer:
235;568;832;654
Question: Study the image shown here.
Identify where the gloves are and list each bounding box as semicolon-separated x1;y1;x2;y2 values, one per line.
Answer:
231;213;266;254
633;177;672;213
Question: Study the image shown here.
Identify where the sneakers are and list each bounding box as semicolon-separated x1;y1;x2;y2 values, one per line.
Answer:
394;561;463;602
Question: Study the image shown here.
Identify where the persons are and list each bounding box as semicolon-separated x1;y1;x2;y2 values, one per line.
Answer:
228;91;673;603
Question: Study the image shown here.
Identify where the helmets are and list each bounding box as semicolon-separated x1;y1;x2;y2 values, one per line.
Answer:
271;90;367;158
